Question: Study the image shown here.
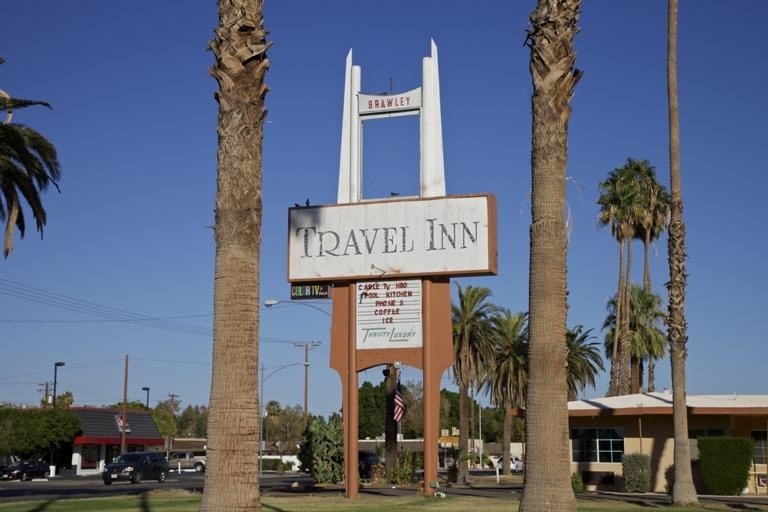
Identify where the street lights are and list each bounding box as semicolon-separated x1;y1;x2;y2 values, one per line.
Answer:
141;386;150;411
262;297;331;318
259;361;312;477
52;361;65;409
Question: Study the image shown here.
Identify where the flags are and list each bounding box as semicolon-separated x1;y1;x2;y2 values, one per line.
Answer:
392;378;407;423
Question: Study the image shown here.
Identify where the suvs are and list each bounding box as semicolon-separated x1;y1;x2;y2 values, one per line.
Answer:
358;450;378;479
100;450;171;485
162;449;207;472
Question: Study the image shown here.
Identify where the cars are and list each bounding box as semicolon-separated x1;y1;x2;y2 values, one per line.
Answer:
496;454;523;474
0;457;50;483
